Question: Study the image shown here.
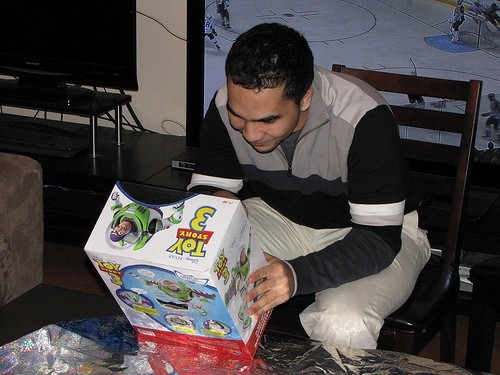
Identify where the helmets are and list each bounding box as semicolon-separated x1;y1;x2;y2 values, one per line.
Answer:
488;93;496;100
206;13;212;19
456;0;462;5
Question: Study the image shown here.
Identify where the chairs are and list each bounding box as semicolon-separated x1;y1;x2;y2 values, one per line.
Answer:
332;64;482;354
464;256;500;374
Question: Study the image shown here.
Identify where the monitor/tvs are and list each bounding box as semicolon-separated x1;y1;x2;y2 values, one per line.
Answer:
186;0;500;223
0;0;139;103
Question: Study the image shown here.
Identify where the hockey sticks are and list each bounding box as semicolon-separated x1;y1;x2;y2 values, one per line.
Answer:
431;17;456;26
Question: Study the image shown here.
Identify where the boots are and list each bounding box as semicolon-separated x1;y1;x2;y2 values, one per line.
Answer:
482;130;490;138
226;23;231;28
451;31;460;43
221;20;226;26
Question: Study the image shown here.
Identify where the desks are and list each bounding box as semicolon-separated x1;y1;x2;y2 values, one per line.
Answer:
0;315;477;375
0;117;199;249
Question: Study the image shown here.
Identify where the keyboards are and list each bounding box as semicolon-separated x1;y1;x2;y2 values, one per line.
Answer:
0;121;93;159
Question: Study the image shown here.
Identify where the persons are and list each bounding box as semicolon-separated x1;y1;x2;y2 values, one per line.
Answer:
406;72;424;108
216;1;232;29
481;93;500;144
204;13;220;51
485;3;500;31
447;0;465;43
187;24;432;349
469;0;487;25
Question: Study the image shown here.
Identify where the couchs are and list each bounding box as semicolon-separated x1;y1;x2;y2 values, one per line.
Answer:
0;153;43;307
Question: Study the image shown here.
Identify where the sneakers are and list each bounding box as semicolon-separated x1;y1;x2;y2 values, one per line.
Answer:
216;45;221;51
